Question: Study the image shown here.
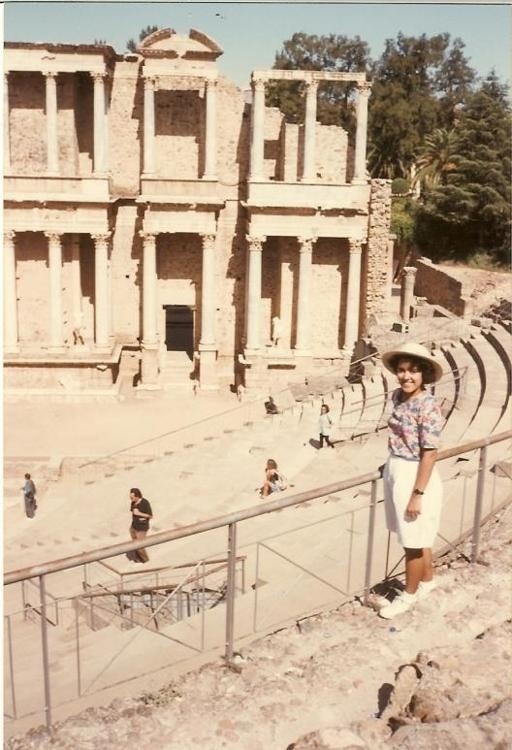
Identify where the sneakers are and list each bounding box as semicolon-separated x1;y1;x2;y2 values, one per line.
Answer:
379;579;436;619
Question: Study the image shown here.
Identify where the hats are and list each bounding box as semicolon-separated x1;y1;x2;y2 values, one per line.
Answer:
382;343;443;384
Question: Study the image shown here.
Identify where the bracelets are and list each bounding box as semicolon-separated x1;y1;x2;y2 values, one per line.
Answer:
412;488;424;495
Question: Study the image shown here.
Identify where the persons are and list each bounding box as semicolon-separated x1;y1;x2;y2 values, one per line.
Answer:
317;404;335;448
128;487;153;564
21;473;36;519
265;469;288;493
378;342;448;620
261;458;281;499
265;396;279;415
